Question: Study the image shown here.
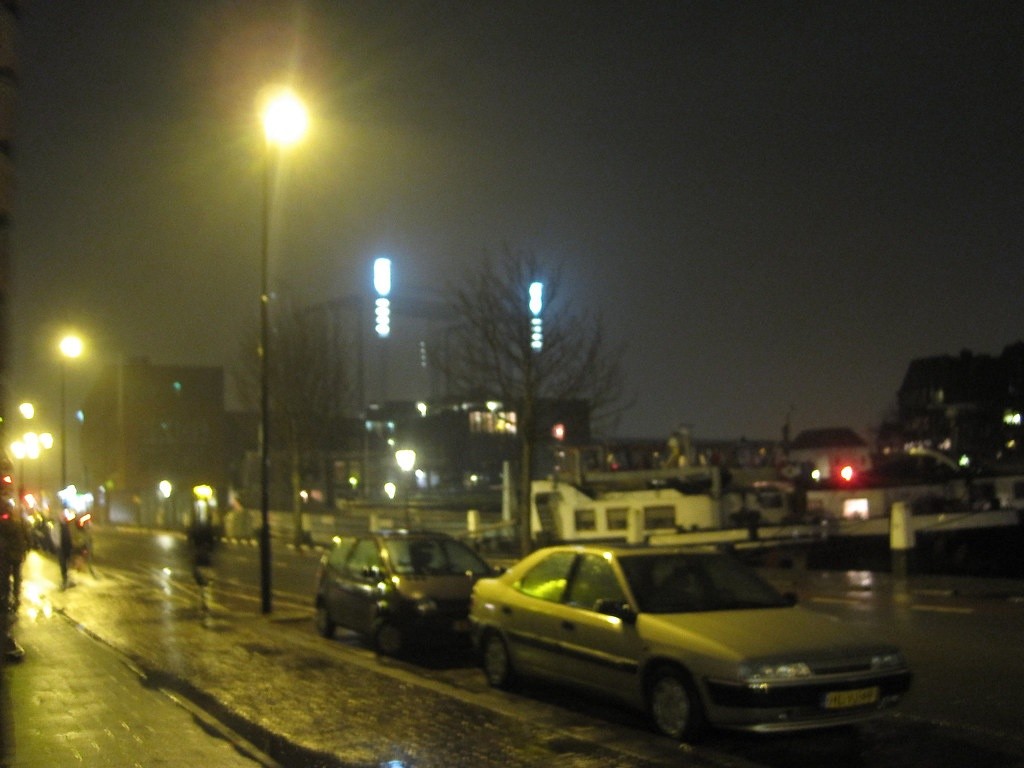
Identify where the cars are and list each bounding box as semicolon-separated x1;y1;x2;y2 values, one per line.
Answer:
466;538;914;746
313;524;508;661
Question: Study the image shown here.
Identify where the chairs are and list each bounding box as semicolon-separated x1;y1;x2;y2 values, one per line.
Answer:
664;565;718;613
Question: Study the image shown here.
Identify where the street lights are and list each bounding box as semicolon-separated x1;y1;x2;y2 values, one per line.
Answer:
17;399;37;526
56;331;85;582
393;447;416;524
251;91;309;616
